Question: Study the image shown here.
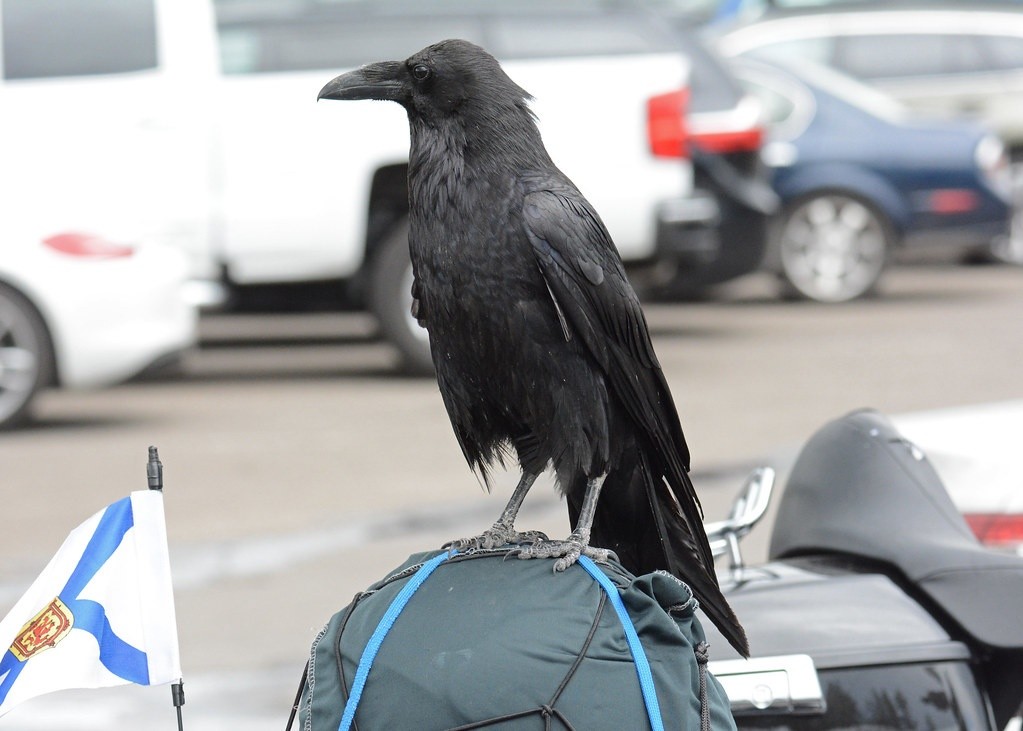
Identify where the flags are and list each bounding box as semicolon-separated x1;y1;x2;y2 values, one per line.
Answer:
0;490;181;717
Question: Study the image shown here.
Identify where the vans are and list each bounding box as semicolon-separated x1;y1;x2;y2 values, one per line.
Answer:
1;6;694;378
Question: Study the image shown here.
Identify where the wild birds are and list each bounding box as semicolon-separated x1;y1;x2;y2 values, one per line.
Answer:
315;37;750;655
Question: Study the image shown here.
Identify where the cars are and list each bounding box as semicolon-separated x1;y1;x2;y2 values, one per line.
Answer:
622;52;791;310
726;11;1023;143
732;59;1018;310
0;143;210;431
